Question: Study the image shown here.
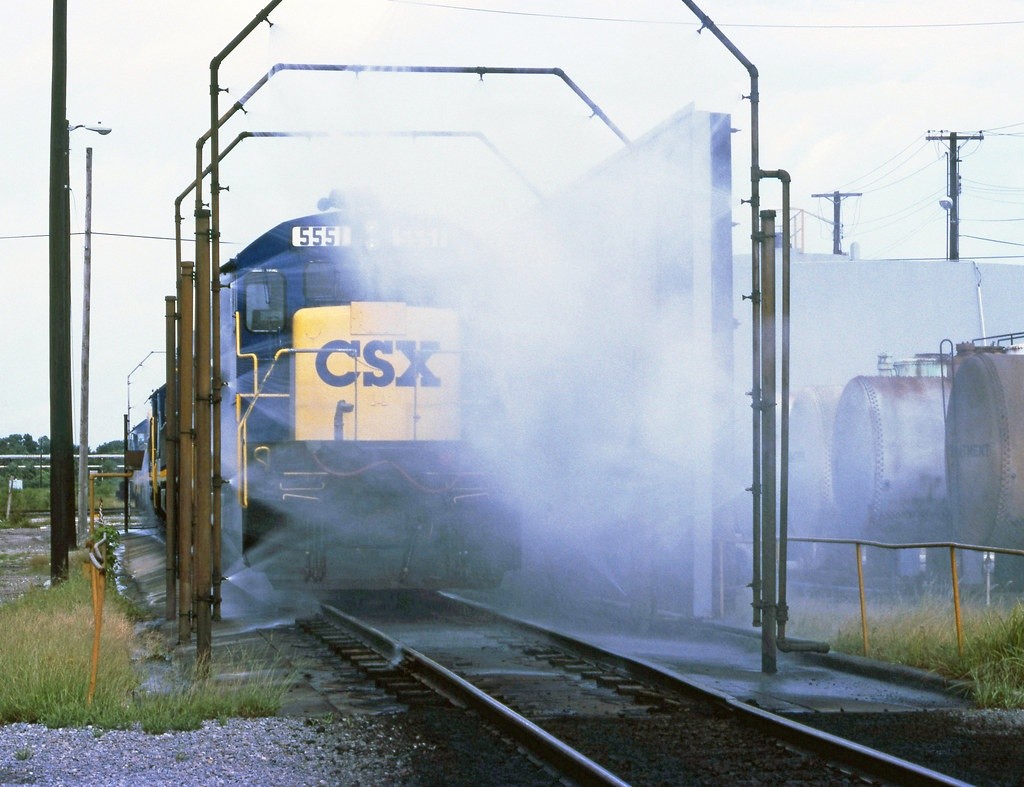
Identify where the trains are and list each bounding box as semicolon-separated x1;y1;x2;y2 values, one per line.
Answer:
128;186;526;594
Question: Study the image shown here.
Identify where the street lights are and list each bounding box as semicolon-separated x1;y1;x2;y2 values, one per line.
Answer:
939;196;954;260
48;118;114;584
